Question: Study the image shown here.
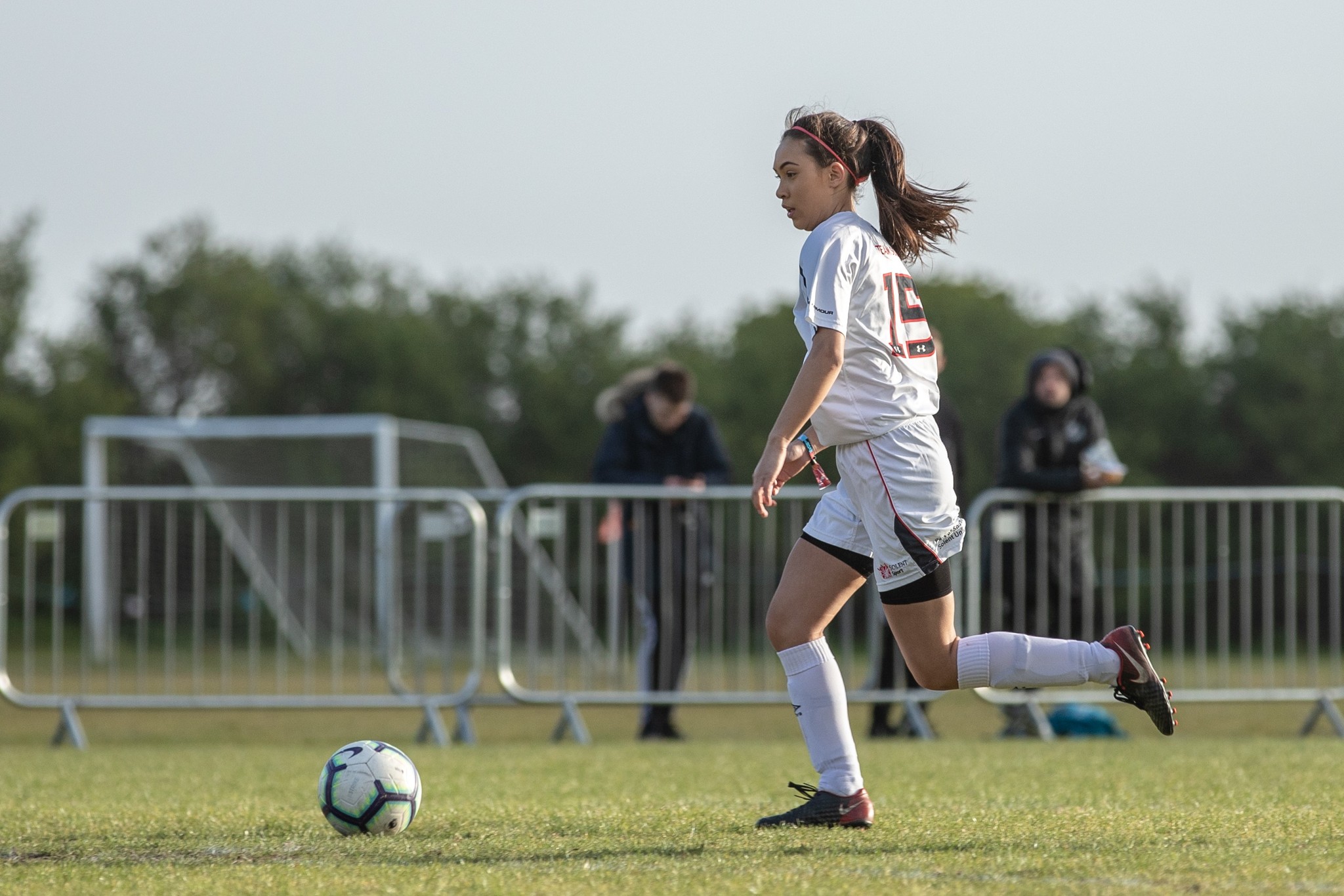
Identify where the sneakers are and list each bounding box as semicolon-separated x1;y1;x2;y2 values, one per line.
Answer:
756;782;880;832
1099;624;1178;736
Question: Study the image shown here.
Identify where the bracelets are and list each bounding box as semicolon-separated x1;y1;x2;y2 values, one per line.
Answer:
798;434;831;490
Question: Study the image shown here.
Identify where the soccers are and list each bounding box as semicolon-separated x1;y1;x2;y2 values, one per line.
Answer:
317;740;421;837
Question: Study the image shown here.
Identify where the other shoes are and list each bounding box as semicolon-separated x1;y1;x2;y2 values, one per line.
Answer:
871;717;903;739
635;718;685;742
907;722;936;738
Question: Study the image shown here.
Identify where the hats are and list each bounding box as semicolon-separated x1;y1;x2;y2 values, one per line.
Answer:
1028;347;1080;393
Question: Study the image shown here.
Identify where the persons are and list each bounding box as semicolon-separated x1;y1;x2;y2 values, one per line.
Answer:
594;359;735;739
748;106;1178;828
989;346;1127;733
869;321;970;740
